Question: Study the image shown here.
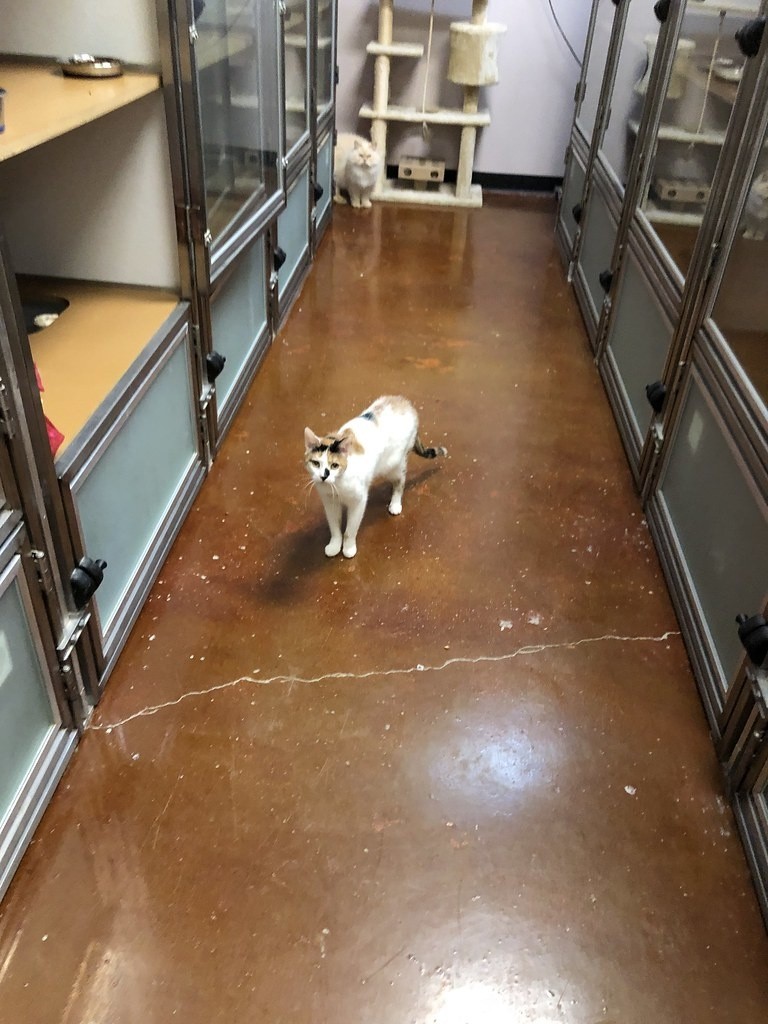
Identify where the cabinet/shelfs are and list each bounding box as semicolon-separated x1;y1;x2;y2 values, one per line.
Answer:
0;0;340;907
557;0;768;936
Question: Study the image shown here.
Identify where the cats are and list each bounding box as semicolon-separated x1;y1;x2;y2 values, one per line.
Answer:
304;394;448;559
333;133;381;208
